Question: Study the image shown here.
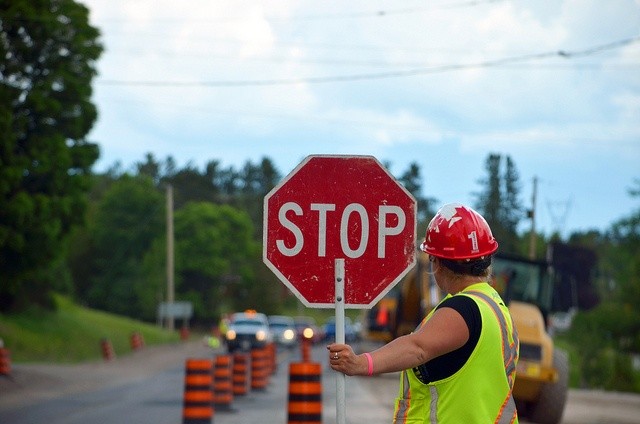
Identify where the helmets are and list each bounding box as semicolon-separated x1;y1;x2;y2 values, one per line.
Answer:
420;203;498;260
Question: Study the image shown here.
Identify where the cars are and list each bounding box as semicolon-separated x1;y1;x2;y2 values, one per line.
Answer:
226;309;272;350
295;315;324;342
266;315;297;347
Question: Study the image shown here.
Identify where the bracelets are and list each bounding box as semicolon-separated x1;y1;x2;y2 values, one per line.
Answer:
362;352;373;376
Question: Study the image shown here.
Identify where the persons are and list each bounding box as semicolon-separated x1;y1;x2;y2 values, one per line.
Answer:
326;202;520;424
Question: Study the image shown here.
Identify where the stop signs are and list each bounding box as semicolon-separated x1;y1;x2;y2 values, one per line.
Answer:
262;153;417;309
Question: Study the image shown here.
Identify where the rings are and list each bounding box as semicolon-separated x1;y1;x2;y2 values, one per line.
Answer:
334;353;338;360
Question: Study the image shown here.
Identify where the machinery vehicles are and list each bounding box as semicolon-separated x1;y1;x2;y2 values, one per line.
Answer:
364;247;569;422
